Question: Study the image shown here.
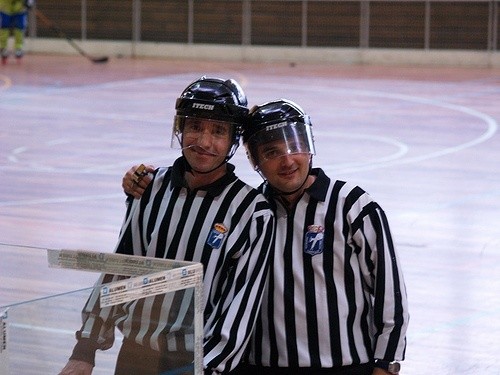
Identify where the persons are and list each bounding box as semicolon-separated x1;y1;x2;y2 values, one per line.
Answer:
57;76;276;375
0;0;52;58
119;99;410;375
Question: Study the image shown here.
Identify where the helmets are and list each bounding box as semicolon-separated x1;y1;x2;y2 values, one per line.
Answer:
175;75;249;144
243;100;312;168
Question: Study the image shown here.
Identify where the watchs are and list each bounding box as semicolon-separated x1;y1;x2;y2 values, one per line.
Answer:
374;359;401;374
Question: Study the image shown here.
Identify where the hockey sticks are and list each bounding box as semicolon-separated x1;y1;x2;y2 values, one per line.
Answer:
28;5;109;64
